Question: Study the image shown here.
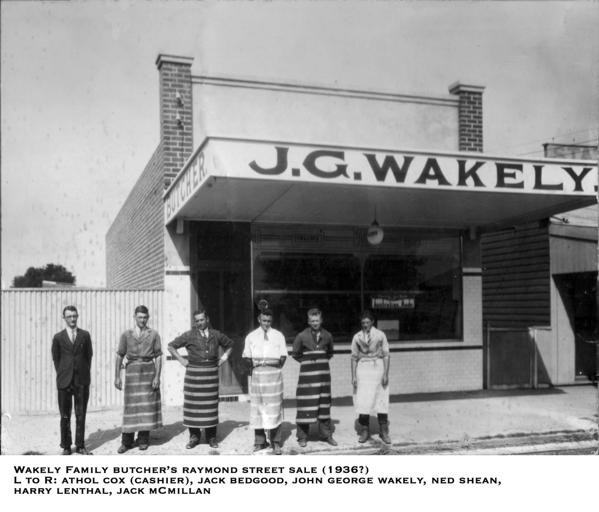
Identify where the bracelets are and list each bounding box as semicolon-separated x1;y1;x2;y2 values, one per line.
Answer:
261;359;265;368
221;352;230;362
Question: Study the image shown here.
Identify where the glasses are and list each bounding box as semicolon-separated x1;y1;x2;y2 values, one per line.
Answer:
66;315;77;318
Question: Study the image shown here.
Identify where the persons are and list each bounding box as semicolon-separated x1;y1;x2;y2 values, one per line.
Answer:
241;309;287;455
290;307;339;447
351;310;392;444
51;306;93;455
168;309;236;449
113;305;163;453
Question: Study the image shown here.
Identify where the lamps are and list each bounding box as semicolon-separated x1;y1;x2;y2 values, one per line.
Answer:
366;202;384;244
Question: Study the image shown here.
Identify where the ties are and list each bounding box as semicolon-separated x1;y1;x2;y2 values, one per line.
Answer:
139;328;142;334
367;331;371;343
264;330;267;340
72;330;74;341
203;330;206;338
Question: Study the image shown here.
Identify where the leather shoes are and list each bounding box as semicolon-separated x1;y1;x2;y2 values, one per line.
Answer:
76;447;92;455
63;448;71;455
254;442;268;451
379;431;391;443
326;437;337;446
209;439;218;447
298;439;306;446
186;441;199;449
118;445;128;453
139;443;148;450
273;443;281;455
359;432;369;442
